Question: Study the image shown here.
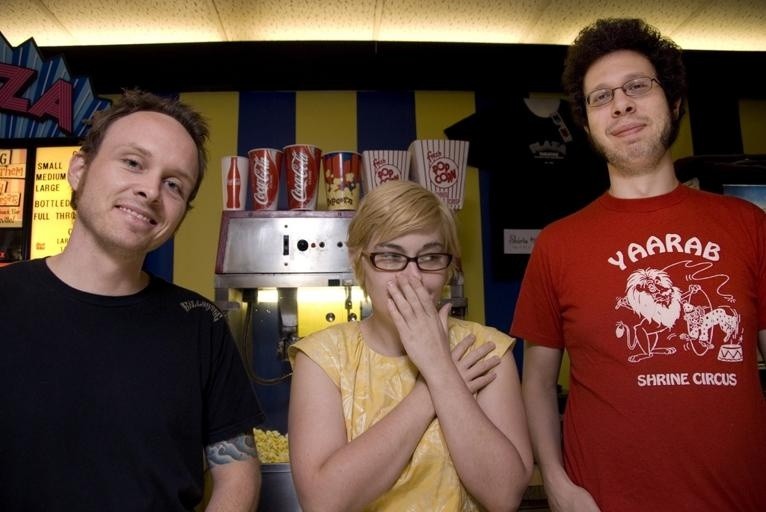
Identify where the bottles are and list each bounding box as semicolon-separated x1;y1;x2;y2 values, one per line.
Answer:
226;157;241;209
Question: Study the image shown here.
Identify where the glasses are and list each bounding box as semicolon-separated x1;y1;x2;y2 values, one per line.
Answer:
587;78;664;106
361;249;453;272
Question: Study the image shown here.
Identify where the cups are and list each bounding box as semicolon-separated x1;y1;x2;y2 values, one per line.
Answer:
322;150;363;211
248;148;283;211
284;144;322;211
221;155;249;213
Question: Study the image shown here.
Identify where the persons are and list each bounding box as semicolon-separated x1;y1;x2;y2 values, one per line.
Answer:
510;18;766;509
285;182;534;509
0;92;266;509
